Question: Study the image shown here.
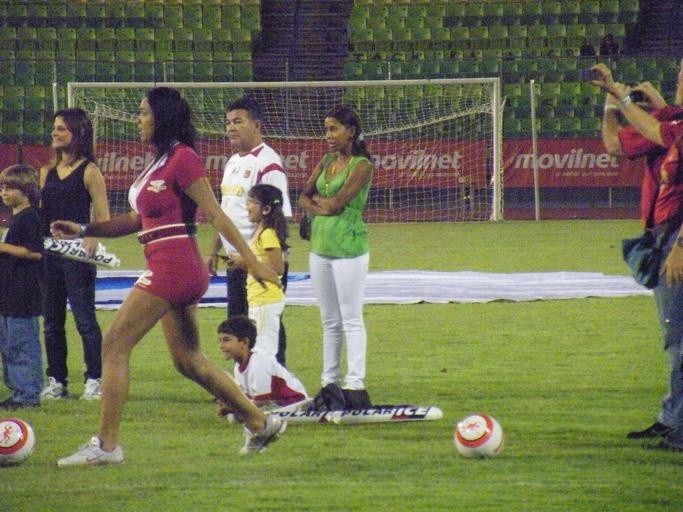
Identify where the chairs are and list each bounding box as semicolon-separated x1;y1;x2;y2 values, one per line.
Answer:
0;0;261;144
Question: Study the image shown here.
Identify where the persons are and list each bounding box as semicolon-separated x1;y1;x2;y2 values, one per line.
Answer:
225;184;292;357
40;108;111;401
216;316;309;424
297;106;375;392
588;62;683;451
50;86;289;468
202;97;294;367
0;164;46;410
602;81;683;234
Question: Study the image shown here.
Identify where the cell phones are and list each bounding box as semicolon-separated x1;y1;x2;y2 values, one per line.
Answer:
579;68;599;82
629;90;647;102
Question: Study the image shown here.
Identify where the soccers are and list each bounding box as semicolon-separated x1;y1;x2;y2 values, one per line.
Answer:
0;418;35;464
455;414;504;458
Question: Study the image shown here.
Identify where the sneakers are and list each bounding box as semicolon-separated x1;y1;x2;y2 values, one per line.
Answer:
40;376;69;398
627;421;674;438
2;397;39;410
641;428;680;451
56;435;123;467
78;378;101;400
241;413;286;453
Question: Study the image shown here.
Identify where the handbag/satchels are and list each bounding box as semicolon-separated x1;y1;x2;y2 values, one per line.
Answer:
621;232;661;289
300;184;316;239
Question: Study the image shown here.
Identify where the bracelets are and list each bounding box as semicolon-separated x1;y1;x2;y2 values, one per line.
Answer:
675;236;683;247
604;104;619;110
203;253;220;257
81;222;88;238
618;96;632;106
